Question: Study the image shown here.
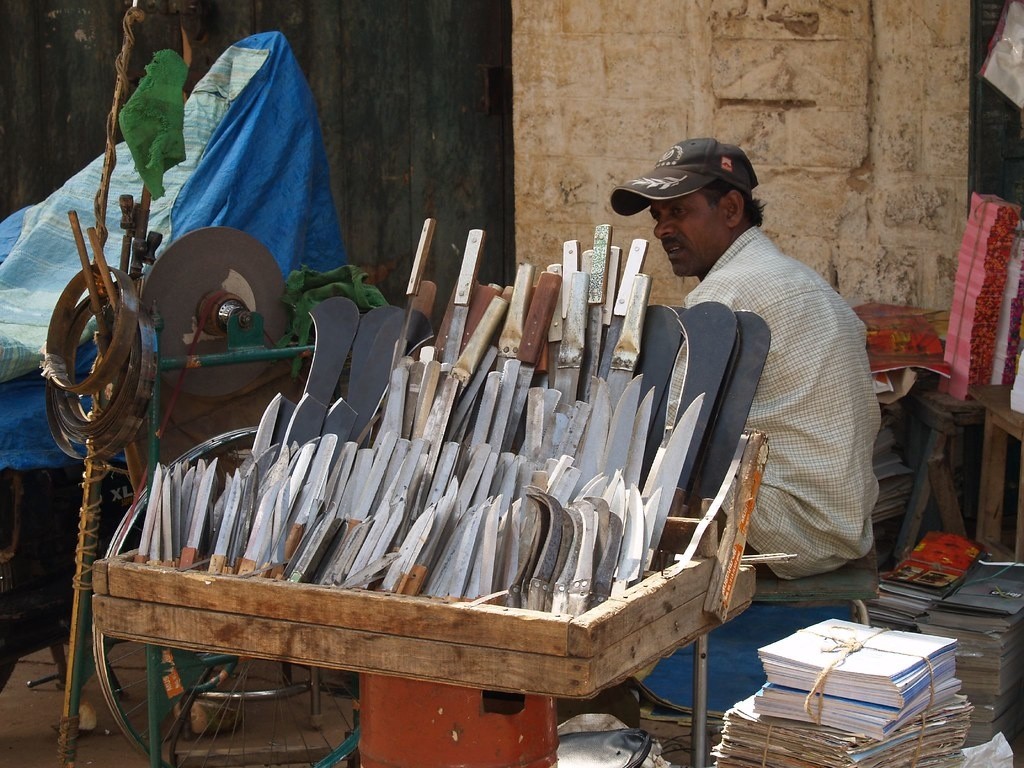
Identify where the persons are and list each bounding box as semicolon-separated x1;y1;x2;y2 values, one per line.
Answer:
610;139;881;580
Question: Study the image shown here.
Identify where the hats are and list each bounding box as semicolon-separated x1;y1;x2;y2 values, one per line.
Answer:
609;138;759;217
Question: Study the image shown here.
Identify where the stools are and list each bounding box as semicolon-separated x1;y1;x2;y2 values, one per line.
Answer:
739;541;878;628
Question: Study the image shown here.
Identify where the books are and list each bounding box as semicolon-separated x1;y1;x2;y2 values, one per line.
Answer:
753;618;961;741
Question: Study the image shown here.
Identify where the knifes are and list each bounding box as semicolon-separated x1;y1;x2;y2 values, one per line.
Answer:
134;219;705;613
